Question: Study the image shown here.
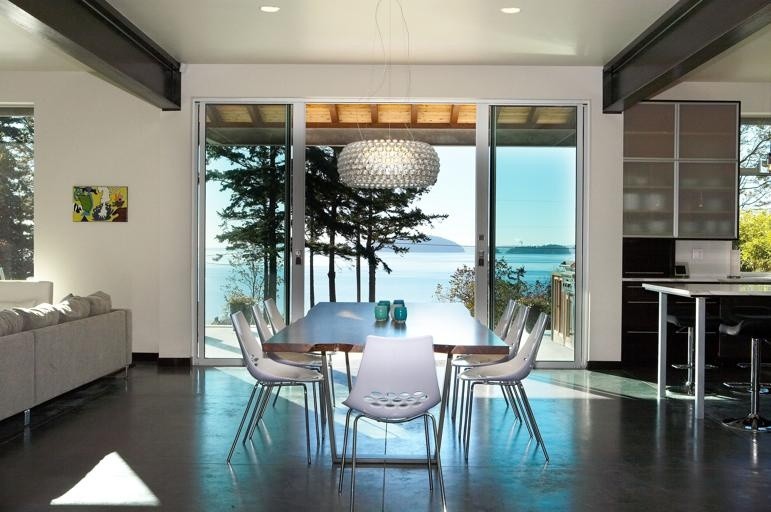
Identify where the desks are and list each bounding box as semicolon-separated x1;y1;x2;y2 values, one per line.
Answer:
641;283;771;420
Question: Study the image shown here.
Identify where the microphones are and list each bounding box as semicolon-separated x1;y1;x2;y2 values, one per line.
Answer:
667;306;771;433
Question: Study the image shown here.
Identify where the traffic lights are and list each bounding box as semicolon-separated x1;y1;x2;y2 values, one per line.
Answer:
0;300;37;311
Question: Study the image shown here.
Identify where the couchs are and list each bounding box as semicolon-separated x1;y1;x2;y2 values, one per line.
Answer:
0;291;133;425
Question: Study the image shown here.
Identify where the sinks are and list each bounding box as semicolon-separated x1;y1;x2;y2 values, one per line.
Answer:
0;291;133;425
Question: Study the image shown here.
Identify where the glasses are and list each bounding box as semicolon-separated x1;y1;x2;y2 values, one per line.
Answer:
337;138;442;189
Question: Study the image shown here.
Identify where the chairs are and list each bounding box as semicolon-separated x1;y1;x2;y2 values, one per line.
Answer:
338;335;446;510
0;280;53;305
227;297;549;464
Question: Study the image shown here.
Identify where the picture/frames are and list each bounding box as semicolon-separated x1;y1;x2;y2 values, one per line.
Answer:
641;283;771;420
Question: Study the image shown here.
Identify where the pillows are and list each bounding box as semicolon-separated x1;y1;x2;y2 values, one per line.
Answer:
0;300;37;311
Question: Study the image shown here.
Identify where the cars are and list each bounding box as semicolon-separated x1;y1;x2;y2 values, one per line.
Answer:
623;167;735;234
391;299;408;324
375;300;391;322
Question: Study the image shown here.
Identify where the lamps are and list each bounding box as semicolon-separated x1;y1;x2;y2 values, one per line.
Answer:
337;138;442;189
0;280;53;305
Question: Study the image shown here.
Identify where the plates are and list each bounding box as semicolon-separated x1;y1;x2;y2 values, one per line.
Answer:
623;100;741;238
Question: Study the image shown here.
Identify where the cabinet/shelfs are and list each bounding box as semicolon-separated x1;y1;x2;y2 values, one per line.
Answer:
623;100;741;238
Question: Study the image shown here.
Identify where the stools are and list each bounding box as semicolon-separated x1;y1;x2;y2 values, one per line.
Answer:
667;306;771;433
